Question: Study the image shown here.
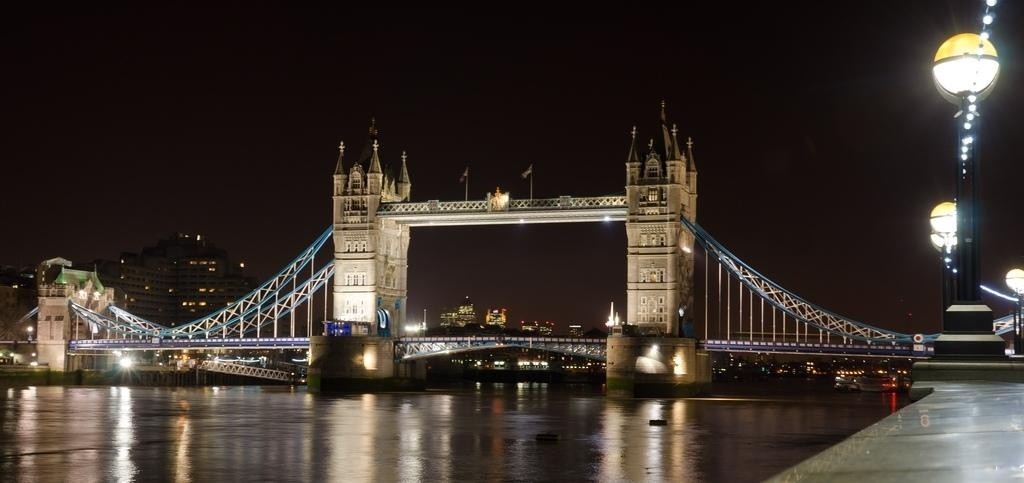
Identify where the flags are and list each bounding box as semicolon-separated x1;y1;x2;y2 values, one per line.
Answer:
521;164;533;179
459;168;468;182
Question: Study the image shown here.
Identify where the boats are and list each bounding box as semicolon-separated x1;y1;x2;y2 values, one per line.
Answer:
832;370;911;393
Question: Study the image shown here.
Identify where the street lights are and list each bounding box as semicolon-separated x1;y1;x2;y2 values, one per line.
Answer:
929;31;1001;303
1004;266;1023;355
928;200;956;358
